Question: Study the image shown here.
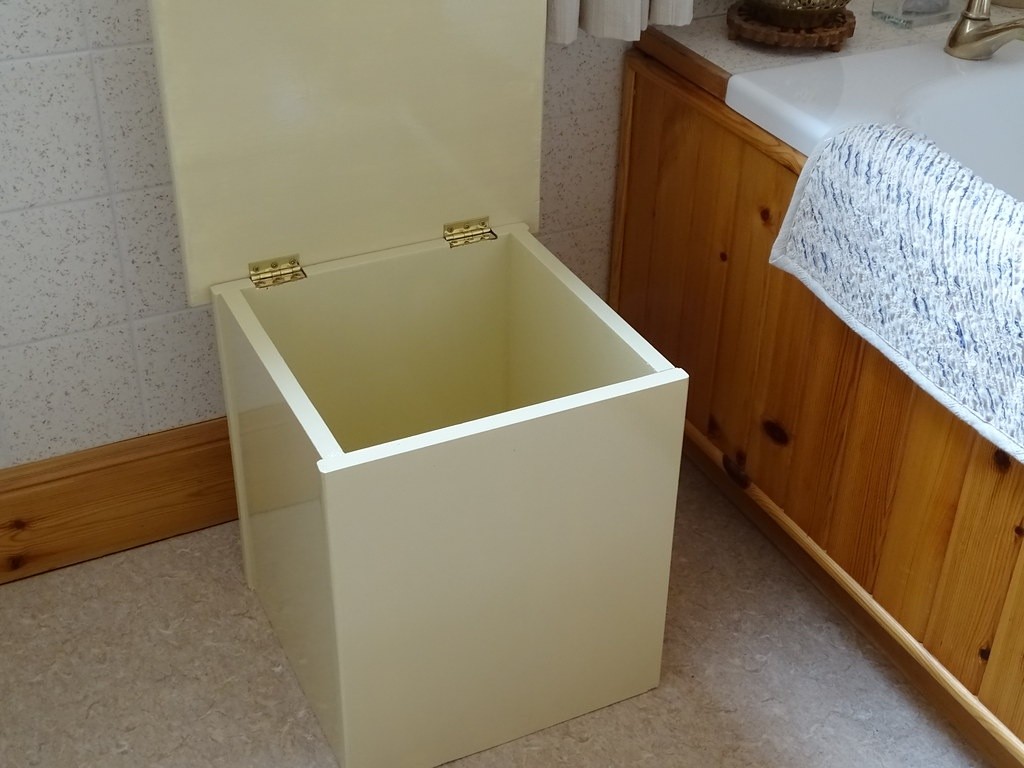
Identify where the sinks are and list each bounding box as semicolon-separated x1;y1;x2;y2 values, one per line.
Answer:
730;37;1024;204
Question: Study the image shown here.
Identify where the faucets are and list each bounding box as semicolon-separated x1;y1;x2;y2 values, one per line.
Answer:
943;0;1024;60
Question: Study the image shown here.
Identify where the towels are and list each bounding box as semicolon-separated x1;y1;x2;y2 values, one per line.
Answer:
768;122;1024;465
546;0;694;46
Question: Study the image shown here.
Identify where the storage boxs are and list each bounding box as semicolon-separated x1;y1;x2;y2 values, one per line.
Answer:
146;0;689;768
